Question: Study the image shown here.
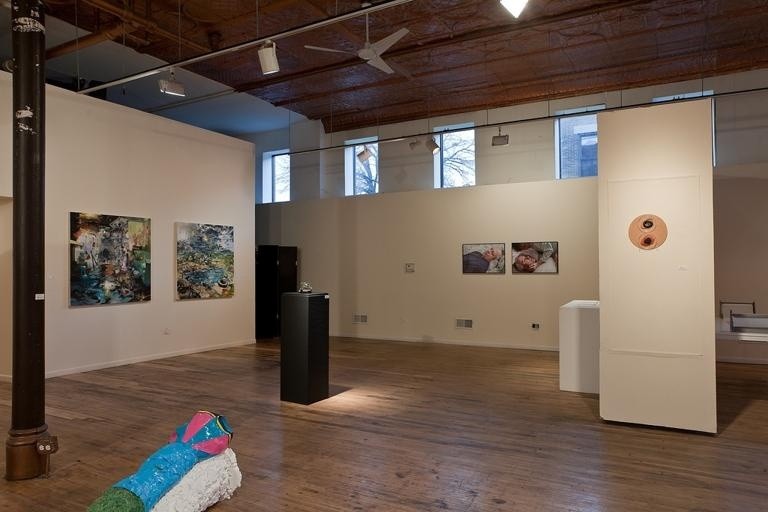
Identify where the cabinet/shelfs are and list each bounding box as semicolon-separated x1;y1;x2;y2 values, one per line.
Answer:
558;298;601;394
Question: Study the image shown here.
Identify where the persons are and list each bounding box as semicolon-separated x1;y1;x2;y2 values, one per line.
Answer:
511;241;558;271
462;244;504;274
511;252;557;273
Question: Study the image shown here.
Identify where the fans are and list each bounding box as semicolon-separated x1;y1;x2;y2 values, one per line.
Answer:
303;2;410;74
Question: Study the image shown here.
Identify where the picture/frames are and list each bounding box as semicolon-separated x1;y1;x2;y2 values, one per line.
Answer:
462;241;559;274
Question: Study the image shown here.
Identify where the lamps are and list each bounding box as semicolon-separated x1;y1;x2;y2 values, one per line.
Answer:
357;125;509;163
156;66;185;97
258;40;280;76
